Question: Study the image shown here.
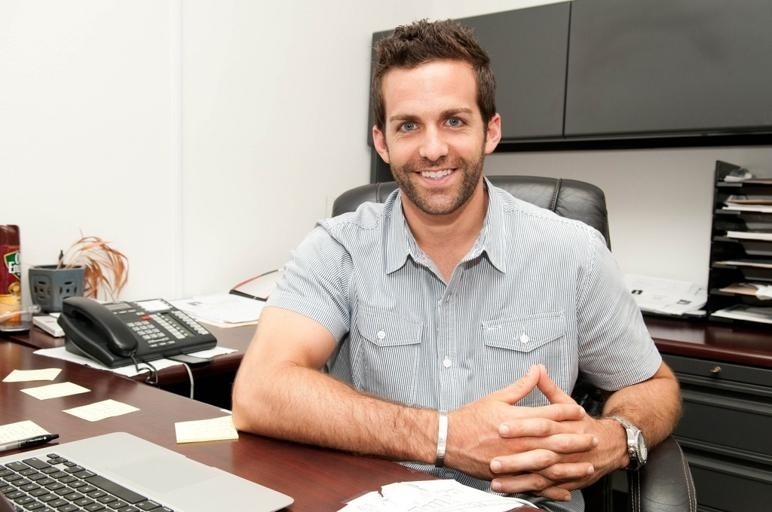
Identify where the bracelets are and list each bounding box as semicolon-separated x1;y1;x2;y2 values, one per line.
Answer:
435;408;448;468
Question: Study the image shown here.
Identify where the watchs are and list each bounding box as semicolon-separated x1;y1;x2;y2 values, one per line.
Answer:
607;414;649;471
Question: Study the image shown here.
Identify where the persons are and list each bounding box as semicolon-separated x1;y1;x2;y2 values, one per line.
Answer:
232;17;683;512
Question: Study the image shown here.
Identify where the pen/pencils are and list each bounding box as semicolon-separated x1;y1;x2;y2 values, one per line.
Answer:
0;433;59;453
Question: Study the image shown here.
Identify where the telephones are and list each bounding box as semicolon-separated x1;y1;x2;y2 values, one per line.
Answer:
58;297;217;368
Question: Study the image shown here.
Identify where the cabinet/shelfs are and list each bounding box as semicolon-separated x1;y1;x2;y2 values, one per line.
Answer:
365;0;771;148
660;351;772;511
702;159;772;331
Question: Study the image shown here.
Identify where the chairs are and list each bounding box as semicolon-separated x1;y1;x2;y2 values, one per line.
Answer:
328;172;699;512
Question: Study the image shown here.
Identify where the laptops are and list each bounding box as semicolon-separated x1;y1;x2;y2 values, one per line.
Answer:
0;430;296;512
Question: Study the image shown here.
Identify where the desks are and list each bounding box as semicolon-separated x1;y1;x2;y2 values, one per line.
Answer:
1;340;536;512
0;290;265;409
